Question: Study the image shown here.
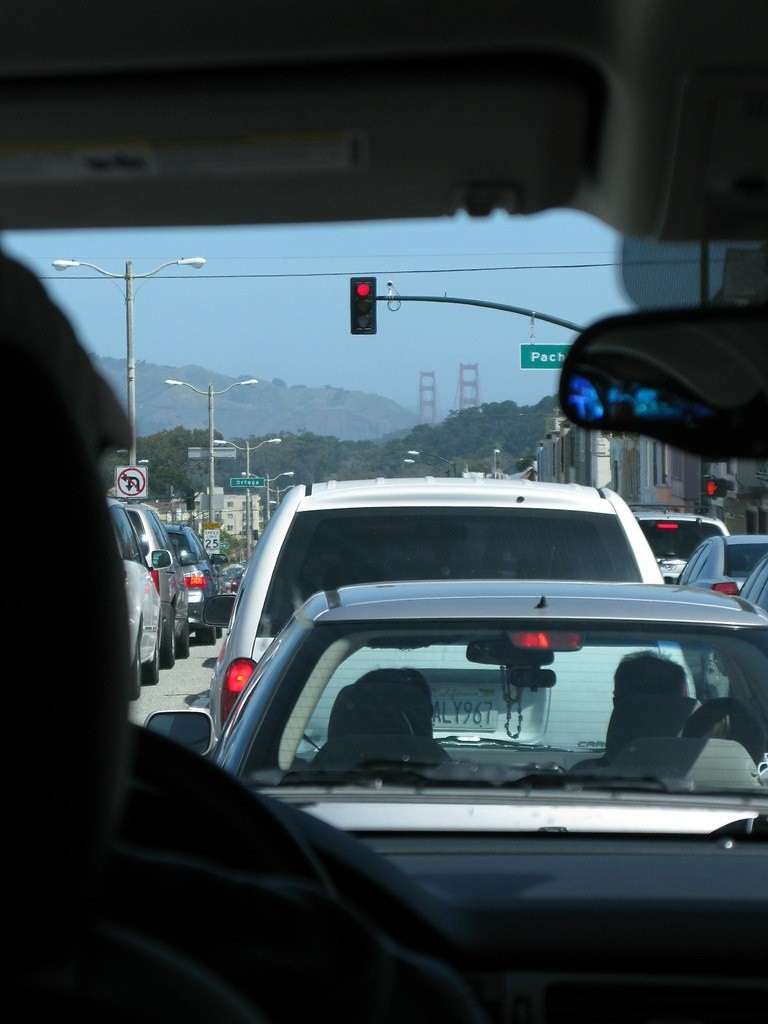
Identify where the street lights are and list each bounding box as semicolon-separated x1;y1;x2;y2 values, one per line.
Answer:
494;448;500;479
163;379;260;521
52;255;207;462
214;438;282;560
404;459;450;477
409;450;457;477
241;471;295;523
257;485;297;508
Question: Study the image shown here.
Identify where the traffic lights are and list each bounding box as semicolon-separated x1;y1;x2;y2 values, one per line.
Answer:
349;277;379;335
704;478;720;497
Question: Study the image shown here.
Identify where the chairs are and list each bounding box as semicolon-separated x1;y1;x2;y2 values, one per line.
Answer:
0;350;475;1024
572;696;705;770
304;734;454;779
662;535;697;560
612;736;762;789
313;684;443;736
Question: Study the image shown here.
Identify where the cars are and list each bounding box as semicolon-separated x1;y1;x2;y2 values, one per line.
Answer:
141;576;768;985
104;496;229;700
702;551;768;616
663;534;768;694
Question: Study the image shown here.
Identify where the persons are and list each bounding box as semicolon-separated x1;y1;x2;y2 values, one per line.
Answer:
0;251;489;966
567;319;763;425
565;652;690;783
362;668;434;741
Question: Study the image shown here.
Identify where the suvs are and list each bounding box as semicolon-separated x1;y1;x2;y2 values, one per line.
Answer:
201;472;699;776
624;508;731;587
